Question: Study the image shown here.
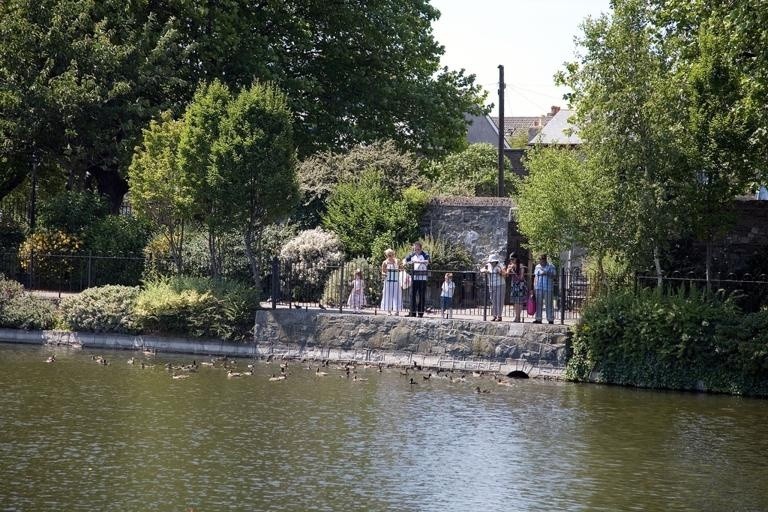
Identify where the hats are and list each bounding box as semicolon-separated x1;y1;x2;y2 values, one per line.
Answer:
486;254;499;262
508;252;518;259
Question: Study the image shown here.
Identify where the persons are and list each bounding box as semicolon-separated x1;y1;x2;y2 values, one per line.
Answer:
381;249;404;316
507;251;529;322
440;272;456;318
347;271;366;313
480;254;507;321
402;243;430;317
533;254;556;324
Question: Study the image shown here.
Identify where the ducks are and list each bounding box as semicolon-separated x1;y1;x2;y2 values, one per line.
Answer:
43;342;258;380
265;355;511;396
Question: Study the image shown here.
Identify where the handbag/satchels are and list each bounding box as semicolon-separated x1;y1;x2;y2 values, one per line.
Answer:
527;300;536;315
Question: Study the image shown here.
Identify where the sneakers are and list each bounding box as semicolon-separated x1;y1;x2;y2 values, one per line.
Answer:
388;312;423;318
353;310;361;314
491;316;553;324
